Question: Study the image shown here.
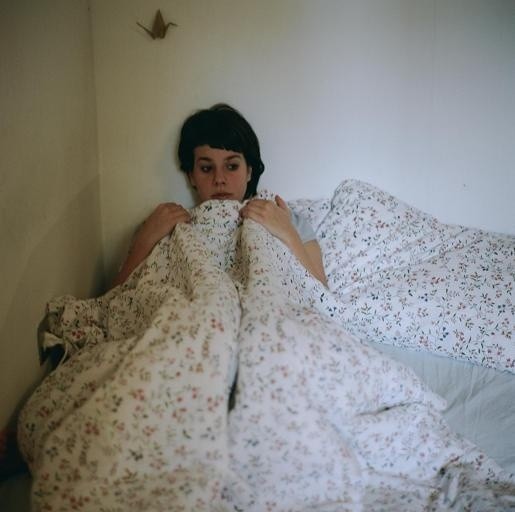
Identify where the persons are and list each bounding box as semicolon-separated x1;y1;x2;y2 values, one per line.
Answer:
111;103;328;290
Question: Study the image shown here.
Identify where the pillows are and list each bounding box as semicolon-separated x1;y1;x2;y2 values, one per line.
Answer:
316;178;515;375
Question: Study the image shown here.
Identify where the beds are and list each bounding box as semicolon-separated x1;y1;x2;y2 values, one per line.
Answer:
16;178;515;510
